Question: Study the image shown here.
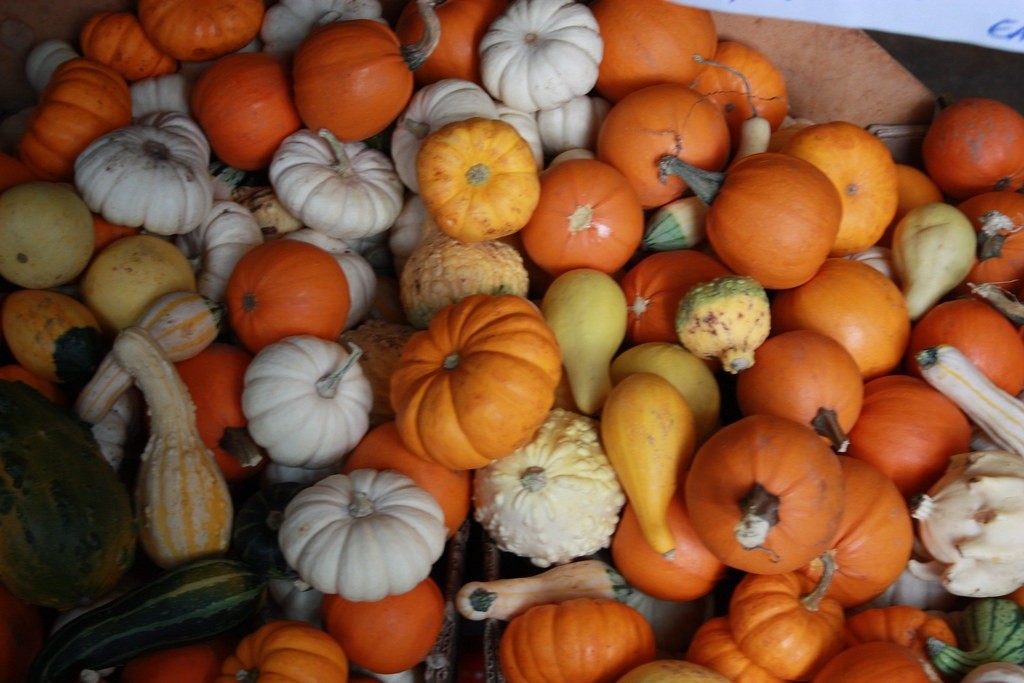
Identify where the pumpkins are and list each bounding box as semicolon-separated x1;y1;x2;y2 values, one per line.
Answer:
0;0;1024;683
